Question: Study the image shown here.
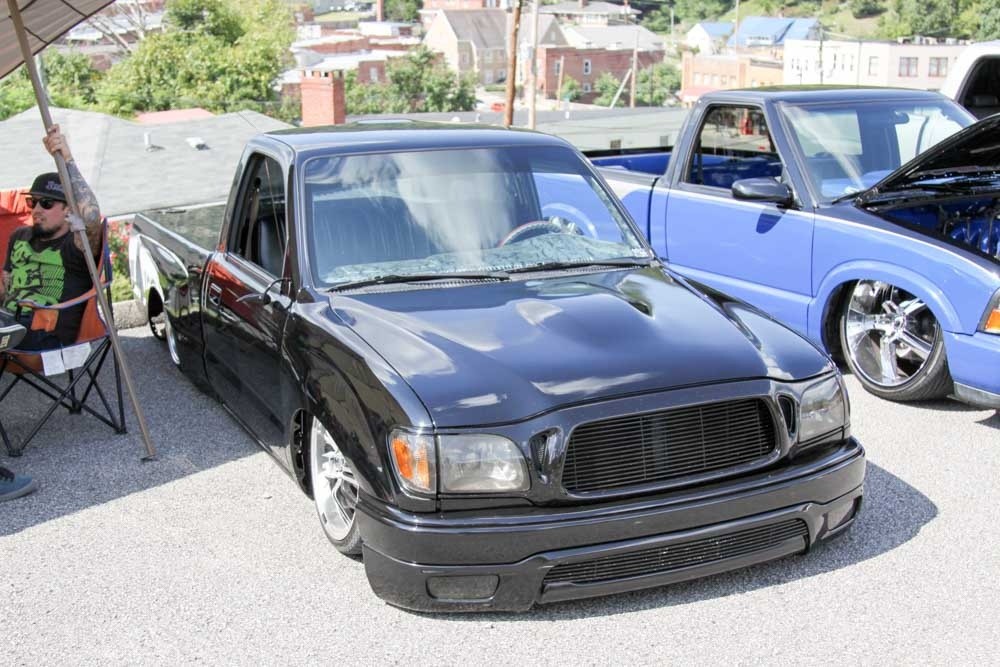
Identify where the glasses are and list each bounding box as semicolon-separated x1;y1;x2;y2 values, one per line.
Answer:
25;197;59;210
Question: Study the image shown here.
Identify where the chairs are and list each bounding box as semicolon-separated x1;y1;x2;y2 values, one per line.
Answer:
0;215;127;457
972;96;998;112
690;159;863;188
256;213;286;277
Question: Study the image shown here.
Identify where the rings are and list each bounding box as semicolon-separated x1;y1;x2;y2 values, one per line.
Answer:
57;137;62;144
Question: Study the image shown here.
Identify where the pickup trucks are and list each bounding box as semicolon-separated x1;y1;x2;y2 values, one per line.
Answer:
127;118;867;615
523;83;1000;412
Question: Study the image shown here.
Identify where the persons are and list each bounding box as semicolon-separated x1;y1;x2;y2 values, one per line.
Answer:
0;124;100;351
0;467;38;502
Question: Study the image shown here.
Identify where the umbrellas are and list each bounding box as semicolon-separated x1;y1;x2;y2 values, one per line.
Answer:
0;0;157;461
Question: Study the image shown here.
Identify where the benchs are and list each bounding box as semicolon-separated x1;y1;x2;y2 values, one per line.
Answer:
589;153;726;175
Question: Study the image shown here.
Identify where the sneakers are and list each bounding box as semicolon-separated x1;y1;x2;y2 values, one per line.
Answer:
0;311;27;352
0;466;33;501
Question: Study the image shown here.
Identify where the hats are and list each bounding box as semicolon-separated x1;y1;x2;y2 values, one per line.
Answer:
20;173;66;202
